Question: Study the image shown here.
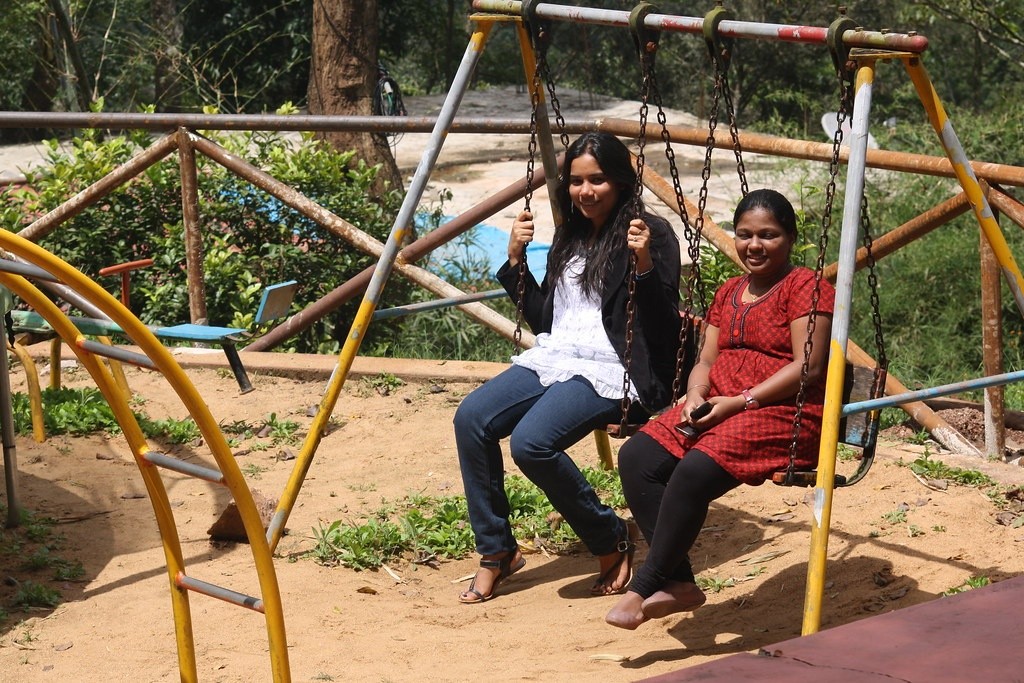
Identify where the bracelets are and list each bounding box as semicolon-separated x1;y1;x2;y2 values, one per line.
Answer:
686;384;711;394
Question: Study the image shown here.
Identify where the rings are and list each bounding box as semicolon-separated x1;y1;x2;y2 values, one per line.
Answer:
634;236;638;241
638;230;642;235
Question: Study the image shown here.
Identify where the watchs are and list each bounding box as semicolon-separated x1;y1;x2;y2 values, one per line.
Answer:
742;389;760;409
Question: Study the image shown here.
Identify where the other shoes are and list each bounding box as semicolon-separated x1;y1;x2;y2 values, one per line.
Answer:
642;586;706;619
605;590;650;630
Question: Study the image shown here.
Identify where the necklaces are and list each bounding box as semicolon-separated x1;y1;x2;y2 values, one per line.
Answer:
747;262;791;301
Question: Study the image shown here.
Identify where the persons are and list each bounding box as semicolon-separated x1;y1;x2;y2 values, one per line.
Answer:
456;129;681;602
604;189;835;629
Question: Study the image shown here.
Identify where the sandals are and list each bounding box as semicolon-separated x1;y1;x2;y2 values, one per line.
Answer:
591;519;639;595
458;547;526;604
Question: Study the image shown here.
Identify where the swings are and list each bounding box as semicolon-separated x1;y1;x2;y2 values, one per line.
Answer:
669;53;889;488
512;41;707;491
